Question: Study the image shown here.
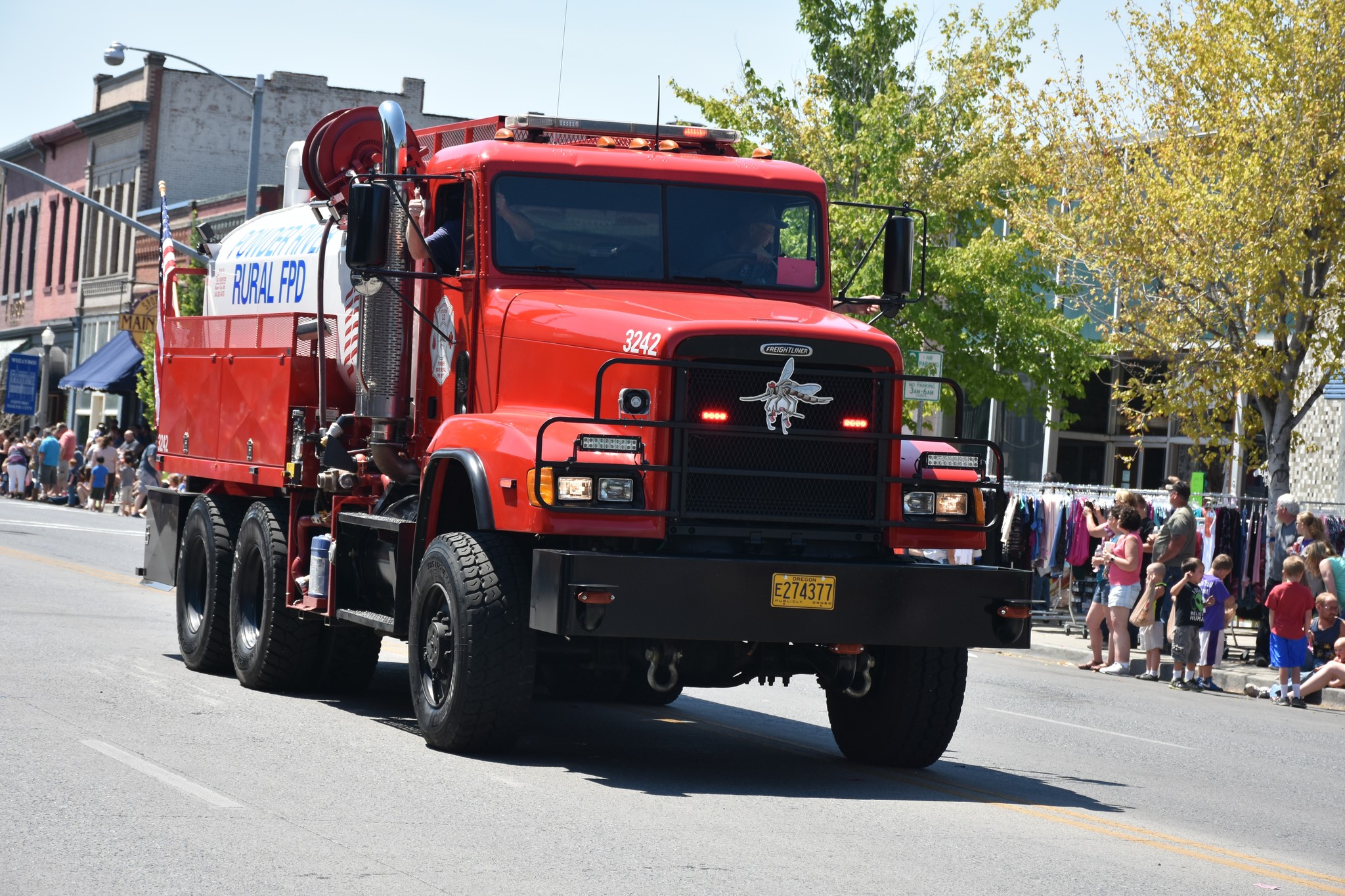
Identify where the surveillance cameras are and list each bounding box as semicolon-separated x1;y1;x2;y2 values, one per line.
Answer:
103;49;124;66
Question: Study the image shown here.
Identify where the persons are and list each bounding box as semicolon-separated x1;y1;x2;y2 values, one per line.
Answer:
702;202;881;315
0;419;187;519
405;192;533;270
1079;476;1345;709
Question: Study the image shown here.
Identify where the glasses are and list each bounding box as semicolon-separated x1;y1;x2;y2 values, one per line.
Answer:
1274;505;1281;509
1324;607;1339;612
1295;522;1302;526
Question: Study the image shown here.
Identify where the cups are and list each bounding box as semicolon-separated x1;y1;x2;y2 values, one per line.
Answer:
1104;541;1115;554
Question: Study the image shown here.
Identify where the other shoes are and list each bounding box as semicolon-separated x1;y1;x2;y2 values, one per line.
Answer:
1102;643;1109;650
1255;657;1268;667
1087;645;1092;649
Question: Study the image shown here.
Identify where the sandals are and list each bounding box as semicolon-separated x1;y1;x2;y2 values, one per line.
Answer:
1271;696;1280;701
1090;662;1109;672
1292;697;1307;708
1078;660;1103;670
1273;696;1290;706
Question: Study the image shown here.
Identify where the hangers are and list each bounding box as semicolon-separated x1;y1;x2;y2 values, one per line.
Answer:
987;479;1345;527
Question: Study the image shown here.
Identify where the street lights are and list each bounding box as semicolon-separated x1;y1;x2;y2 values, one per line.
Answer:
33;325;55;438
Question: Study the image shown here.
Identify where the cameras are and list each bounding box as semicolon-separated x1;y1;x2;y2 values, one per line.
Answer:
1085;502;1093;511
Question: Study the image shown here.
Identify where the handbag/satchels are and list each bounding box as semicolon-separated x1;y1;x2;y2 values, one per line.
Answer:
1223;603;1238;629
1129;587;1156;628
1167;595;1176;642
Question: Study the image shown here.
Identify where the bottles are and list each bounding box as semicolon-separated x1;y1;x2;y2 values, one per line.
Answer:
1092;544;1102;568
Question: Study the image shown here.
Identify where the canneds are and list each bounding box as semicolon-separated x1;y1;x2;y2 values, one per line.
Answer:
1293;542;1300;553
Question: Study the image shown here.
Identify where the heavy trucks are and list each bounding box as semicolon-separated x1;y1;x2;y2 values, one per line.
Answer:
131;98;1043;766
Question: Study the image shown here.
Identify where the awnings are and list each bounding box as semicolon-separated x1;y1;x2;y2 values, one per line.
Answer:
58;330;148;397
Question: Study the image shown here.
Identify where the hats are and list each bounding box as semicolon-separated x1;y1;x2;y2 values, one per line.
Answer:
1165;481;1190;496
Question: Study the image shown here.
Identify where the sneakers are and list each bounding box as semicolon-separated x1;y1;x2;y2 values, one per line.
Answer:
1140;674;1159;682
1135;673;1148;679
1195;676;1202;685
1184;678;1204;692
1099;662;1117;674
1200;677;1223;693
1243;683;1269;698
1105;663;1130;677
1169;677;1190;691
